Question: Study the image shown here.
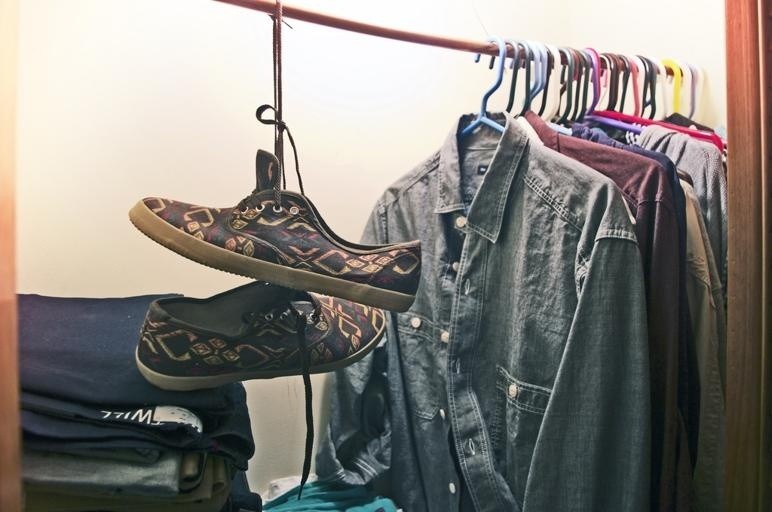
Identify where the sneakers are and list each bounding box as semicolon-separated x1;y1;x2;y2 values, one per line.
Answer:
129;0;422;312
134;281;386;499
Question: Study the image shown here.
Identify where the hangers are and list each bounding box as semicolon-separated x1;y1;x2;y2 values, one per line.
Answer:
458;40;727;165
